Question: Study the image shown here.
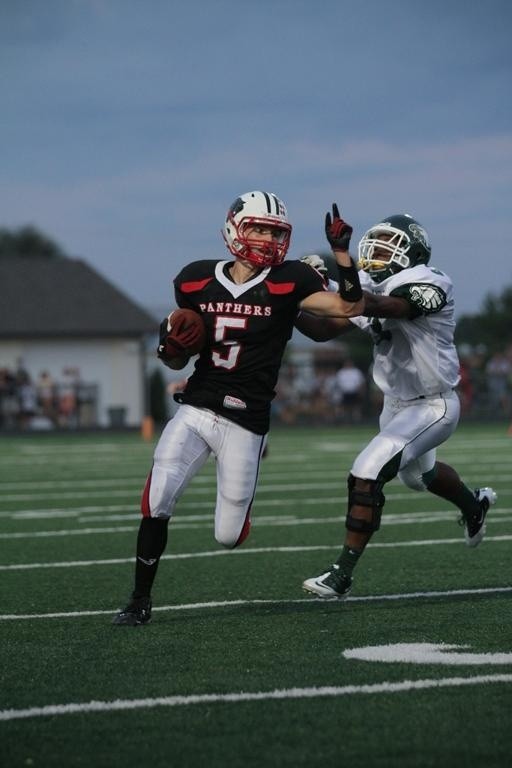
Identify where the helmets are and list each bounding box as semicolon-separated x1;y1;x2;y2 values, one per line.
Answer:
357;214;433;283
221;190;293;268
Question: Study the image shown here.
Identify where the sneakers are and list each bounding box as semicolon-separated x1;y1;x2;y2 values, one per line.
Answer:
301;563;354;600
457;486;498;548
112;589;153;627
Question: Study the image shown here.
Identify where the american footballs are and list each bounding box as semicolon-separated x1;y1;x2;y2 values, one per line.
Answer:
166;308;206;357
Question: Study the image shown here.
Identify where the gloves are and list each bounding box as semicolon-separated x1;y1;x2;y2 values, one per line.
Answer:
324;202;353;252
157;315;203;362
300;252;338;275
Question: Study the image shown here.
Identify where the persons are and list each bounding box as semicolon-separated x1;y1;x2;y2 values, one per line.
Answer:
271;359;365;425
109;190;367;627
456;340;512;420
0;354;89;434
298;214;498;601
